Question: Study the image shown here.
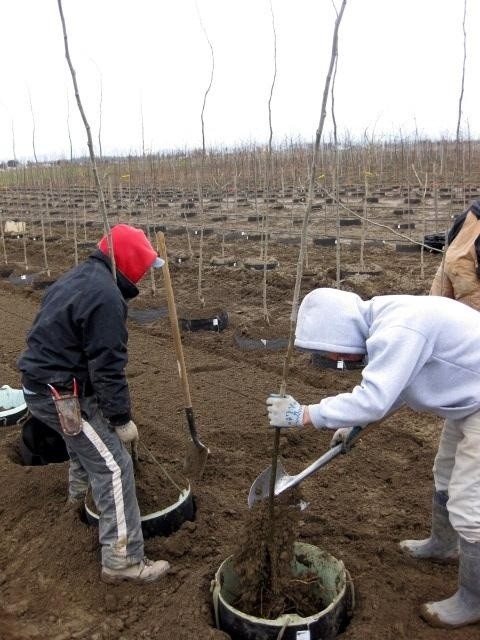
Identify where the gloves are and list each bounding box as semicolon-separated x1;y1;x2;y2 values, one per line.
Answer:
265;393;307;429
113;420;140;442
329;426;361;454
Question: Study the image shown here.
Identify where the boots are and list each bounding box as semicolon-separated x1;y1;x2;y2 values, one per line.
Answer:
419;537;480;629
395;488;459;560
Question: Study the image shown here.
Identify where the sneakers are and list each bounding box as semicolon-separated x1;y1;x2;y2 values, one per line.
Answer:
101;555;171;586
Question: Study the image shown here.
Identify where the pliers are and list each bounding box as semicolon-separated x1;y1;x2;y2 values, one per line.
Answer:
47;377;77;434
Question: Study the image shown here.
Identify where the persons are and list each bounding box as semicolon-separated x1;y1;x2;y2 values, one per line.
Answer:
15;224;171;581
429;198;479;310
266;286;480;627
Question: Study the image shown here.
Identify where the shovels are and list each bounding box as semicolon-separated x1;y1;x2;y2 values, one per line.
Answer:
248;401;408;521
156;231;210;486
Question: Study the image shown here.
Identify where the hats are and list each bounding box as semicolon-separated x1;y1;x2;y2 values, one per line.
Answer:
151;257;165;268
